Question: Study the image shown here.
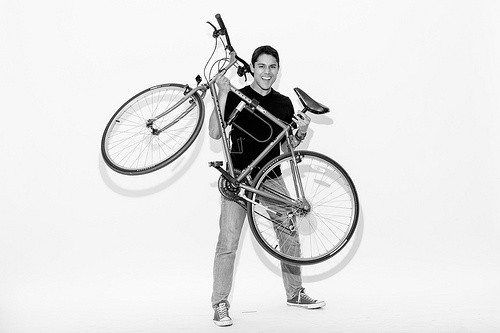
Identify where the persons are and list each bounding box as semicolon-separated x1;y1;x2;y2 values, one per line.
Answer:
209;44;327;326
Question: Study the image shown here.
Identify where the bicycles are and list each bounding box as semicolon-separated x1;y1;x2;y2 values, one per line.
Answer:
100;14;359;266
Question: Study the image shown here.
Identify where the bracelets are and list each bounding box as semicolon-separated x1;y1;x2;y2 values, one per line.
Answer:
295;129;306;141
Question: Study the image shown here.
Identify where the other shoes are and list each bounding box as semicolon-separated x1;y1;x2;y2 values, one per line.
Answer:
212;300;233;326
287;290;326;309
